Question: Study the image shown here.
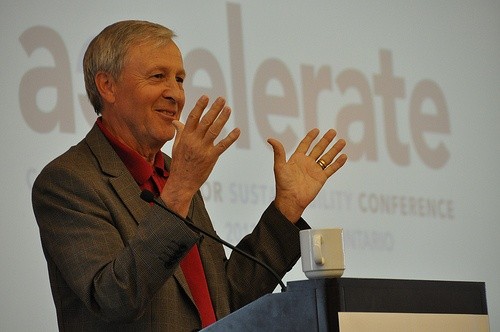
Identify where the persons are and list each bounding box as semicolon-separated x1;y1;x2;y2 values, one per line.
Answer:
30;20;349;332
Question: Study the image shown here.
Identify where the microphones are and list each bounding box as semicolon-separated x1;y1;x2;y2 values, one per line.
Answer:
140;188;287;292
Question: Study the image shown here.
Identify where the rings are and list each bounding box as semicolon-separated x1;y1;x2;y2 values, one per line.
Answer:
317;159;328;168
